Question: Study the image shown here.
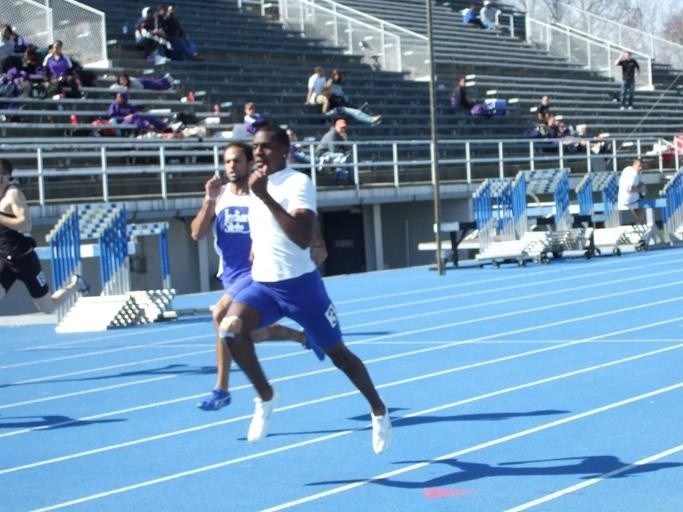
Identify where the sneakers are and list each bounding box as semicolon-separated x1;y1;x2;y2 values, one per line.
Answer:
371;397;391;454
196;389;232;410
302;329;324;361
247;387;278;441
73;274;89;295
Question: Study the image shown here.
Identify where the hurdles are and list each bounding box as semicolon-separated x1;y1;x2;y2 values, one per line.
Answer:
432;169;683;269
46;204;177;334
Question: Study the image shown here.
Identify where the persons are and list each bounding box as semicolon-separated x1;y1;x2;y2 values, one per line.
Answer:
190;141;324;411
448;76;619;155
617;160;646;227
243;101;352;177
461;3;487;29
217;121;394;454
110;73;206;140
479;1;503;32
307;66;382;126
134;4;199;64
0;160;89;313
614;51;640;111
1;25;86;101
